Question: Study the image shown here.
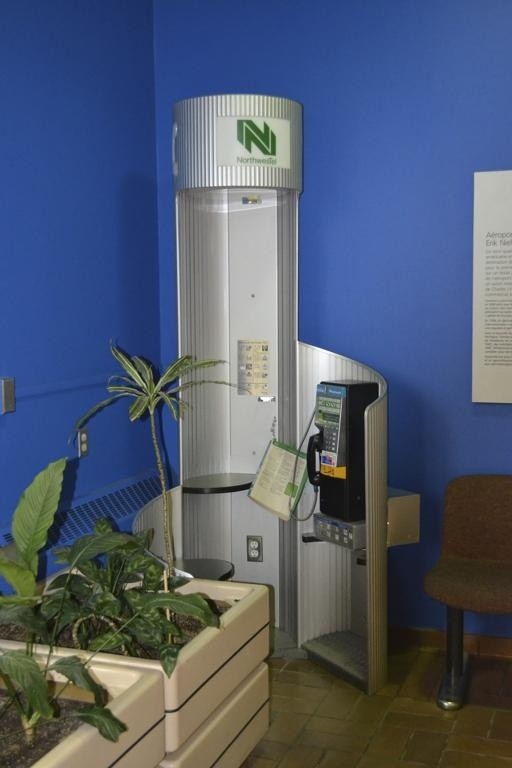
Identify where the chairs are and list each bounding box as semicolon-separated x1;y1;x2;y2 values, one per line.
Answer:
422;474;512;710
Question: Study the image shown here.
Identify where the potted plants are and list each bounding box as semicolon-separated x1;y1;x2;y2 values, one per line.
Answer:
2;337;271;767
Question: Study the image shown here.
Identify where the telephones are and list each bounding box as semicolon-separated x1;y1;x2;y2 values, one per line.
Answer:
306;383;345;484
